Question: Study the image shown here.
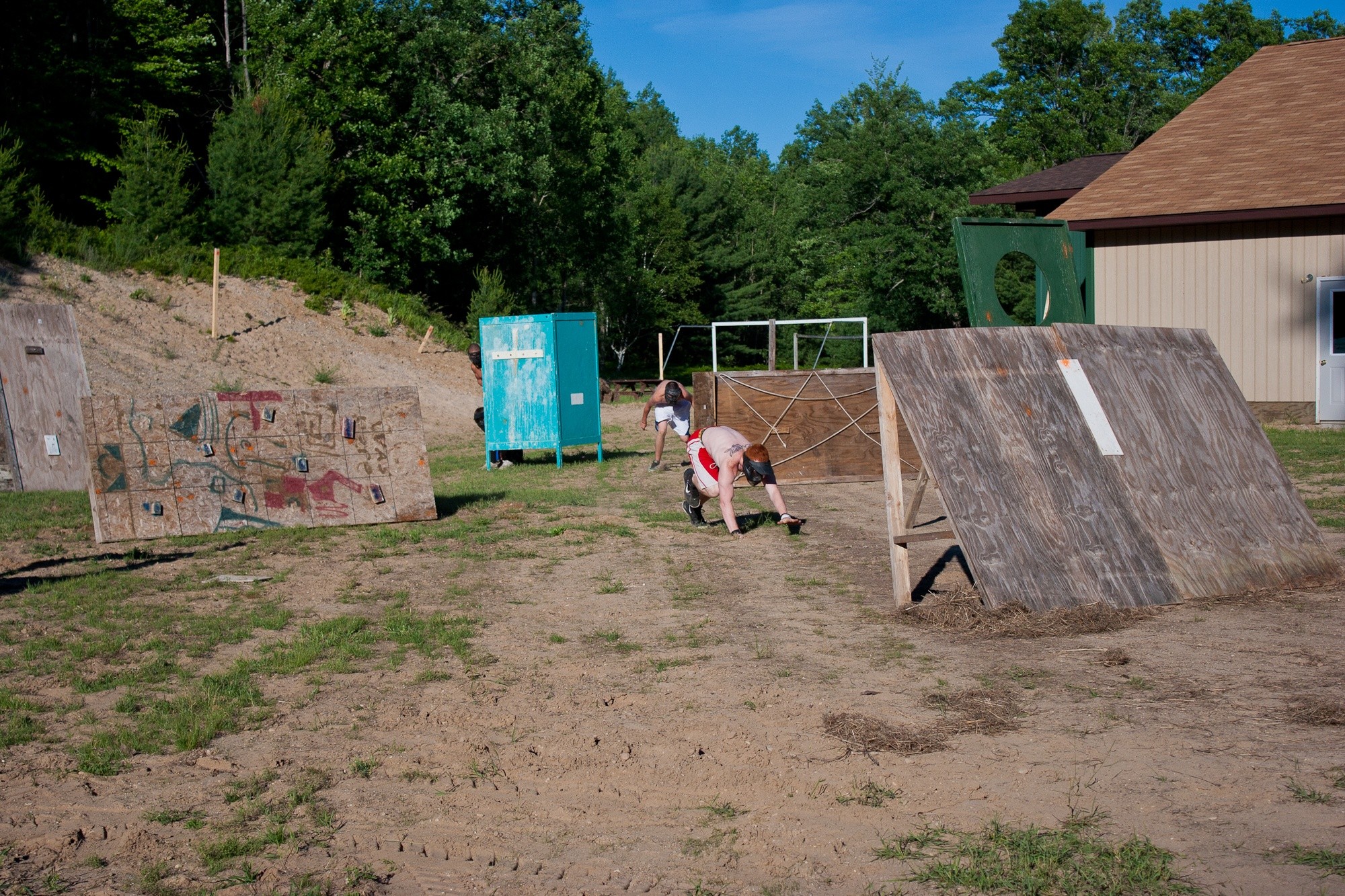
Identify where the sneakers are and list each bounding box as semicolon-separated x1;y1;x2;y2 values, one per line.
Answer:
683;467;701;508
682;501;708;527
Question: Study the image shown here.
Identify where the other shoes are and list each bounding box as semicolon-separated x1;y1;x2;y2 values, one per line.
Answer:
649;459;659;471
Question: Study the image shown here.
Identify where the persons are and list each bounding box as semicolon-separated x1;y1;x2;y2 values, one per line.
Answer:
468;344;514;468
640;379;693;469
681;426;804;538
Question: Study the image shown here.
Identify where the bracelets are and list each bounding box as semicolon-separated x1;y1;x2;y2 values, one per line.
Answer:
641;421;646;424
731;529;741;536
781;513;791;519
